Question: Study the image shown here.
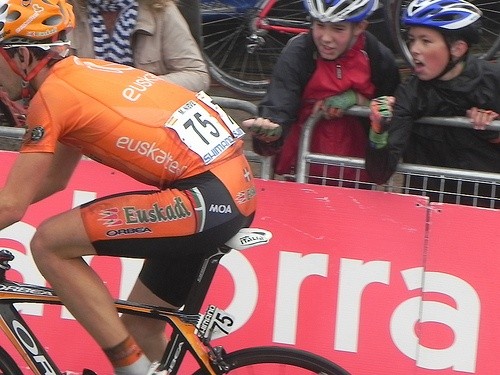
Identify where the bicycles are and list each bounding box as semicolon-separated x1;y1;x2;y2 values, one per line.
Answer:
183;0;500;99
0;227;351;375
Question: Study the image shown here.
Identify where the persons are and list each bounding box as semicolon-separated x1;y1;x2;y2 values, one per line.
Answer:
65;0;210;94
0;0;256;375
242;0;402;190
365;0;500;209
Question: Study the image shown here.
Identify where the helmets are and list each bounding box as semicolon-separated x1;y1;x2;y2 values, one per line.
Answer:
401;0;483;29
303;0;379;22
0;0;77;43
